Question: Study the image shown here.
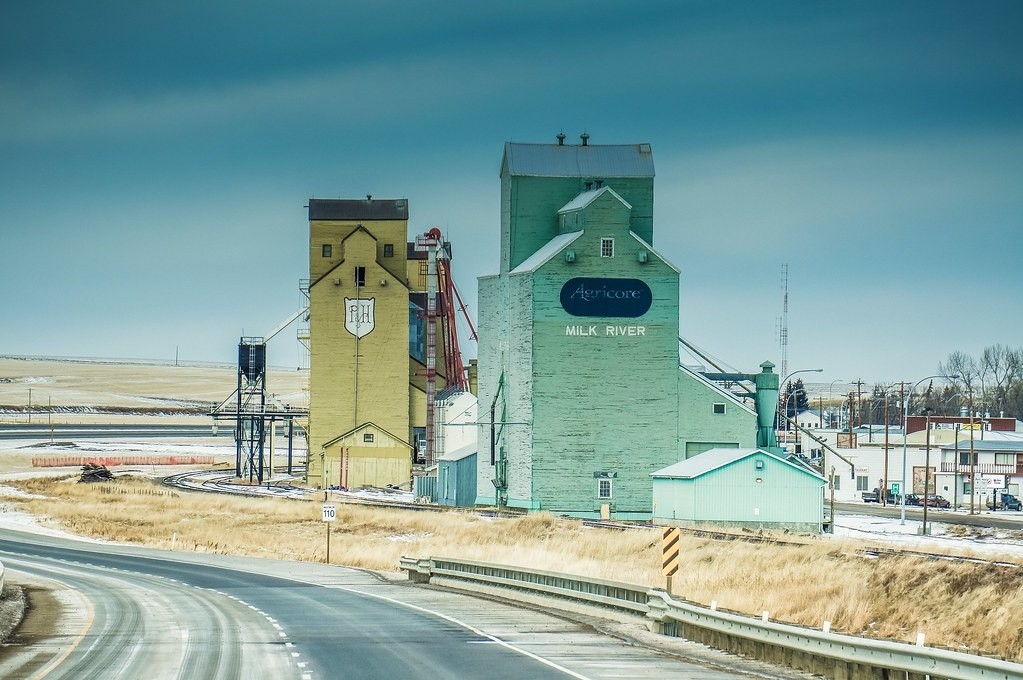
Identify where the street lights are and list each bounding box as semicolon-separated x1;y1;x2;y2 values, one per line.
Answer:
784;388;814;452
829;379;843;429
900;375;960;525
775;369;824;448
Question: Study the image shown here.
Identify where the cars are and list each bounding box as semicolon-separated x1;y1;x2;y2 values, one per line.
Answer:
896;493;920;505
918;494;950;508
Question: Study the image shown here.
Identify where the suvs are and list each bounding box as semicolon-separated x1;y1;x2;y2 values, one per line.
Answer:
985;493;1022;512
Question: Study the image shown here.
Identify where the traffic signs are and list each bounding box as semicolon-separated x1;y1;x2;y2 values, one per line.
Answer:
891;484;900;495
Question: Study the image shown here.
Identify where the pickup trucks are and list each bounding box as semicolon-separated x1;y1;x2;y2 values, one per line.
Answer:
862;488;900;504
